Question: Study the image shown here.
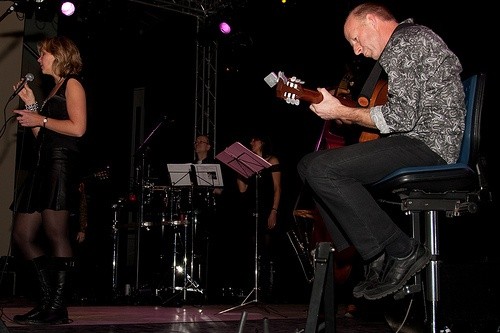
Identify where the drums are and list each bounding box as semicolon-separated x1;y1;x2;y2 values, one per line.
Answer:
117;185;210;235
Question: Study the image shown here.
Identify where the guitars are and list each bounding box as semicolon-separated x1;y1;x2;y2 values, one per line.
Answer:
264;70;389;149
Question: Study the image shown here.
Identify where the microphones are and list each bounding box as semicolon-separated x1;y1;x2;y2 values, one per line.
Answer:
7;73;34;101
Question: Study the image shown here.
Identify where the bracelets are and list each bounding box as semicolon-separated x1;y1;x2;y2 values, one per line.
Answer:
25;101;38;111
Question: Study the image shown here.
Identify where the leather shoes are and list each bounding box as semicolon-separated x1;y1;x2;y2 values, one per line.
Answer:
352;252;386;298
362;237;433;300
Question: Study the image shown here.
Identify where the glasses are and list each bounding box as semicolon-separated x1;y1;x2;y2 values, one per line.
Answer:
194;140;208;145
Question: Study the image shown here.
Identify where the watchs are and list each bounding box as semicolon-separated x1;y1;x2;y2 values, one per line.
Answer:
43;117;48;127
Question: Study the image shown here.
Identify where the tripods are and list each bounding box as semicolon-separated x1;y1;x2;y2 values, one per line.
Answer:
107;142;288;320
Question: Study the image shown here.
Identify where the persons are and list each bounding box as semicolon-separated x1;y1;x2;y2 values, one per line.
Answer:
297;3;467;300
184;136;225;303
237;135;281;303
76;182;88;242
9;36;87;326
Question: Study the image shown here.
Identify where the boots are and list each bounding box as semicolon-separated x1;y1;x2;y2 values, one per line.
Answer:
25;256;73;325
12;254;54;323
